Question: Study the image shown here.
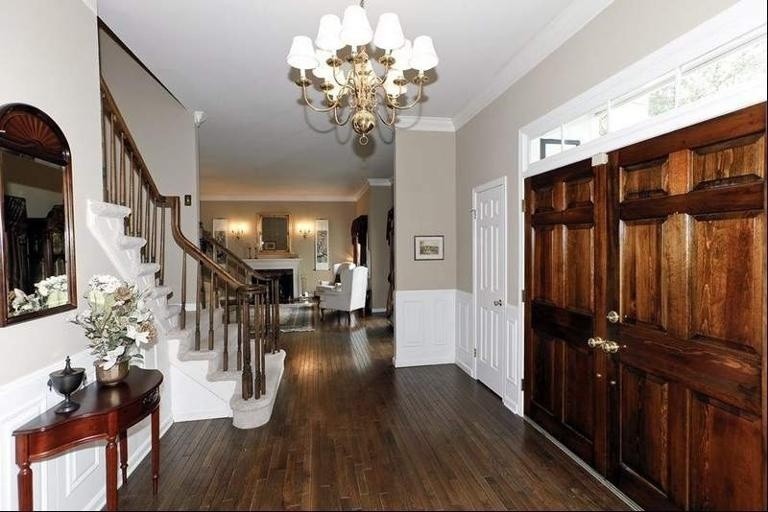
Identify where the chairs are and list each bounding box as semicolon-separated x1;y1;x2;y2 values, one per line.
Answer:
311;261;354;301
320;266;367;324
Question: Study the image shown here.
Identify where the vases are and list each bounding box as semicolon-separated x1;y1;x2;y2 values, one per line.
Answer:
93;360;130;386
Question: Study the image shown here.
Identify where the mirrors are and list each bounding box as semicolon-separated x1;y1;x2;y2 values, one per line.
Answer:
1;102;78;332
256;211;291;253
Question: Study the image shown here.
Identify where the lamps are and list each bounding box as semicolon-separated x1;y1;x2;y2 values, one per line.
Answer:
284;0;441;146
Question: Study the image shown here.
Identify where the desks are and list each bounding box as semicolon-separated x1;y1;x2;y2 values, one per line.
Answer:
5;365;168;510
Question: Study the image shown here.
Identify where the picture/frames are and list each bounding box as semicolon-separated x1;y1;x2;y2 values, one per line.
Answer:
414;235;444;261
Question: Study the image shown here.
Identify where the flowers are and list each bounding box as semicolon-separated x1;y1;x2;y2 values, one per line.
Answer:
70;272;157;364
9;272;70;317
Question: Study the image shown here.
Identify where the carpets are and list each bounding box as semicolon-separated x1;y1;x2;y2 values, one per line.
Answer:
224;302;318;336
